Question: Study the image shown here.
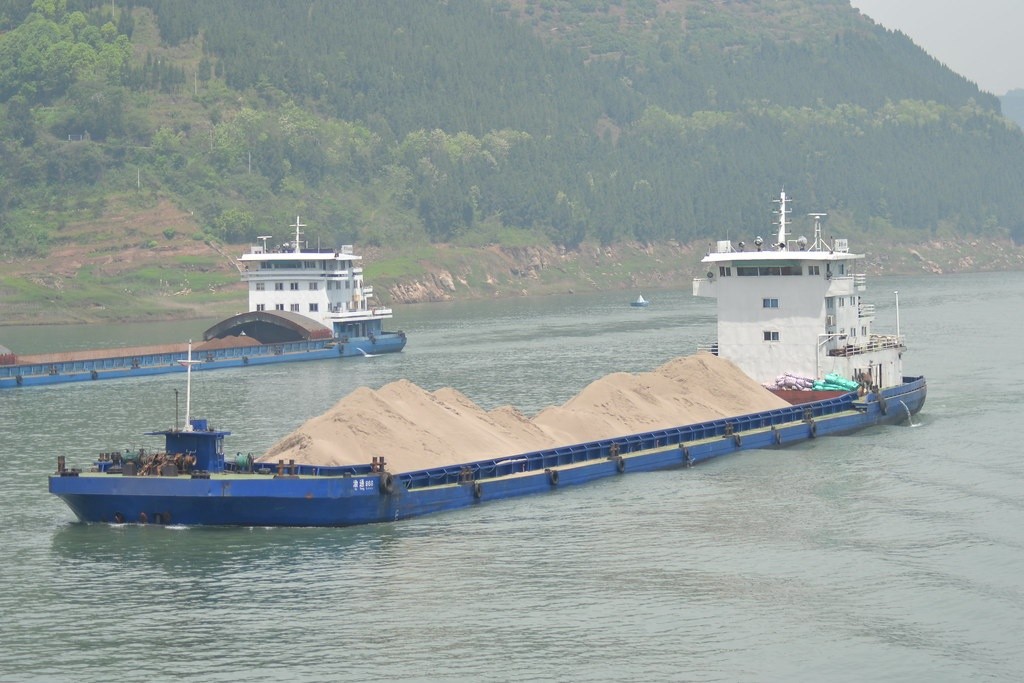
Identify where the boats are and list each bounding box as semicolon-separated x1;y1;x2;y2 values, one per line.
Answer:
1;213;407;391
47;182;928;528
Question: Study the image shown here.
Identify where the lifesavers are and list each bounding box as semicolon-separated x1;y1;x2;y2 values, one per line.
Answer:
379;471;396;496
734;433;742;448
549;469;559;486
878;400;888;416
474;482;483;499
809;422;817;439
339;343;344;353
244;355;248;363
91;370;98;380
16;375;23;385
775;432;782;445
111;510;124;524
683;447;691;461
616;457;625;474
